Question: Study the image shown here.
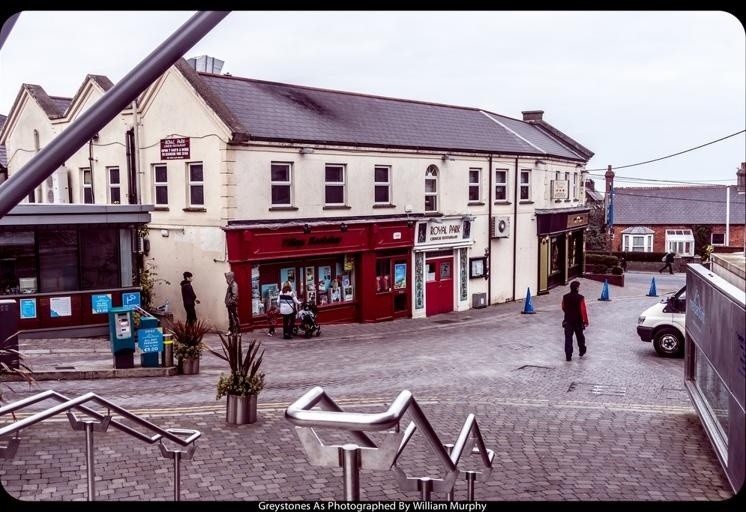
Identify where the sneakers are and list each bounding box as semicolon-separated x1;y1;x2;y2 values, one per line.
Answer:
267;333;272;337
225;330;232;336
579;347;586;356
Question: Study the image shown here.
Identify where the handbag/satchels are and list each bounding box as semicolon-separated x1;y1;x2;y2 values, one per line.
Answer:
293;308;297;315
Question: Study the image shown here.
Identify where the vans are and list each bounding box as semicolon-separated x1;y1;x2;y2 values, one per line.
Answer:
637;283;686;358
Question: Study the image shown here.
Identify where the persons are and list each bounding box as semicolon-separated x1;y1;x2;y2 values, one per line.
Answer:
620;250;627;270
659;252;675;274
296;306;315;329
265;305;281;336
277;281;302;339
224;270;242;337
561;281;588;360
180;271;200;328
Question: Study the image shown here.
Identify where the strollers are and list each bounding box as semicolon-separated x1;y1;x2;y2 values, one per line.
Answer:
292;303;321;339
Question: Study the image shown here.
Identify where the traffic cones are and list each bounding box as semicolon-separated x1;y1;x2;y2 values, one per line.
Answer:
522;288;536;314
599;279;611;301
646;277;659;297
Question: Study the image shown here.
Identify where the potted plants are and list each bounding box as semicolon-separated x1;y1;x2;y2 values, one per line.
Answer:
208;324;267;425
163;317;213;374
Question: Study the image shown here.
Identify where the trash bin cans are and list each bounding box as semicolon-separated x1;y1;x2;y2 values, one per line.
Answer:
160;333;174;367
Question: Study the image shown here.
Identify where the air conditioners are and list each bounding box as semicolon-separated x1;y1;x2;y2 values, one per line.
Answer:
491;216;510;238
